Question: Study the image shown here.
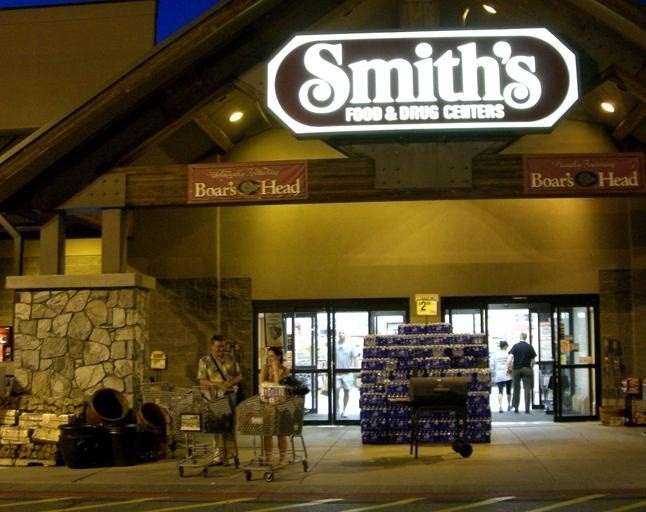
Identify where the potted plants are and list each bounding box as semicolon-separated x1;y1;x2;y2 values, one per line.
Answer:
598;337;626;427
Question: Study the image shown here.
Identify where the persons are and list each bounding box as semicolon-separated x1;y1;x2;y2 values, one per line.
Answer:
258;346;290;469
494;341;512;413
327;331;360;418
507;333;537;413
197;335;244;466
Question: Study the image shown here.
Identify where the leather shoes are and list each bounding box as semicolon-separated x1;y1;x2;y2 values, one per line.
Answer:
224;461;231;465
208;460;223;465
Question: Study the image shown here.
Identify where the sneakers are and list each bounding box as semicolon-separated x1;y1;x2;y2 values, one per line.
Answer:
499;407;529;413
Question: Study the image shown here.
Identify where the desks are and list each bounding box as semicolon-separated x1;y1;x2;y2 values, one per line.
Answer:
410;403;472;459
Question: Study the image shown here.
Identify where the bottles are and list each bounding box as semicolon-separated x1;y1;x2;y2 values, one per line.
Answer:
359;323;492;444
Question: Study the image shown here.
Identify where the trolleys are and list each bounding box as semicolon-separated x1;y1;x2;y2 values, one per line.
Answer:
178;385;238;477
236;394;307;482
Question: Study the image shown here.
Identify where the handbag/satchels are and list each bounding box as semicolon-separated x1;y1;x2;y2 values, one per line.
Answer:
206;396;233;419
320;376;328;396
236;382;245;405
279;377;309;395
